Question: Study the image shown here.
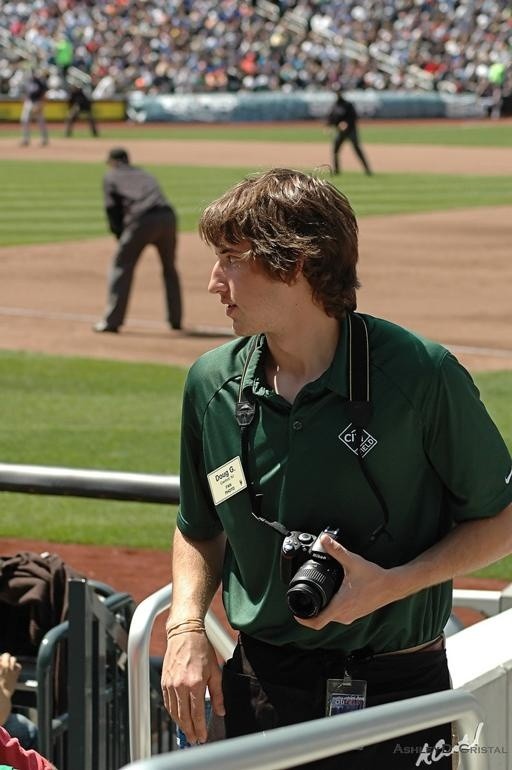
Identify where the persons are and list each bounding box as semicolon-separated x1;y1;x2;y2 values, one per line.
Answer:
160;167;512;770
328;83;371;176
19;69;50;147
0;652;39;752
0;0;512;100
65;84;97;137
92;147;182;333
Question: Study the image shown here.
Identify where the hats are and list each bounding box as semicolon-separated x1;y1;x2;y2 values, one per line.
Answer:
108;149;126;160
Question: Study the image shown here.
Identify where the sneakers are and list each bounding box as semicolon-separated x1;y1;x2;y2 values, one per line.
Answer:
92;323;116;332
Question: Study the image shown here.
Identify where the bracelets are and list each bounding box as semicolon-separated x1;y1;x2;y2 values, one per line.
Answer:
166;618;206;640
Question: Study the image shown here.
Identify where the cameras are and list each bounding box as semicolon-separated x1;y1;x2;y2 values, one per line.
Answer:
281;526;345;619
